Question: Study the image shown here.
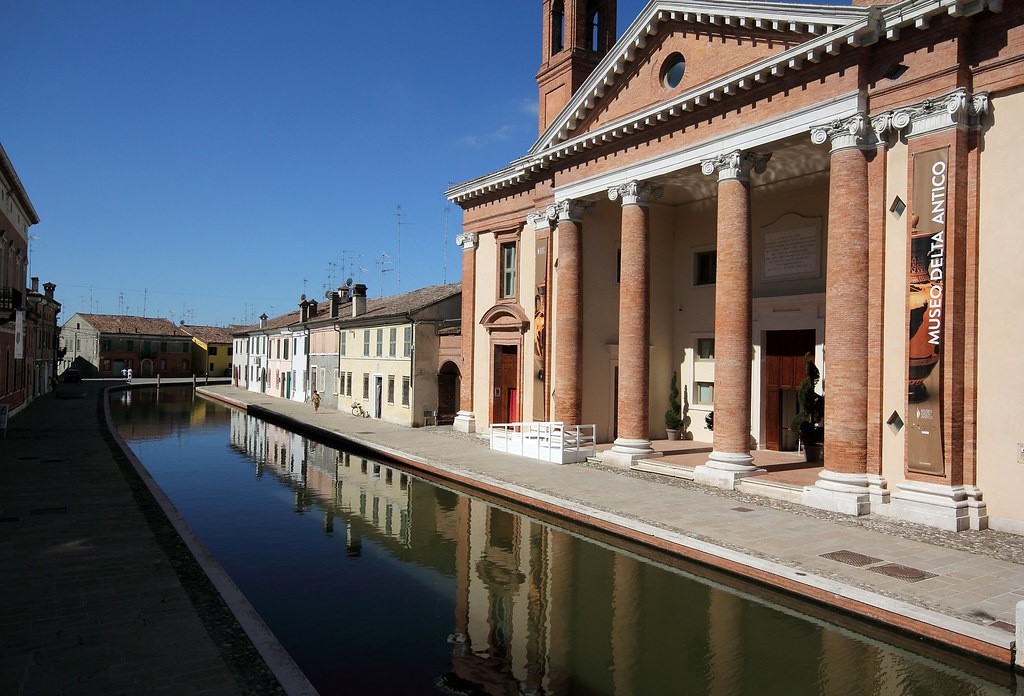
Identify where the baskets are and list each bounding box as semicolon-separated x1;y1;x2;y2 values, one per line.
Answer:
351;403;356;408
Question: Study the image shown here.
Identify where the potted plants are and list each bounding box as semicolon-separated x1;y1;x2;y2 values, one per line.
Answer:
664;370;684;440
790;353;825;464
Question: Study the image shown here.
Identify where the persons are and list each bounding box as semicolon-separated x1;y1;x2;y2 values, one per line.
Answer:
121;368;133;383
48;376;58;392
311;390;322;414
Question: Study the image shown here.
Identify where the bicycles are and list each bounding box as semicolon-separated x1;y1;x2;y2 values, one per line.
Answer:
351;397;365;418
